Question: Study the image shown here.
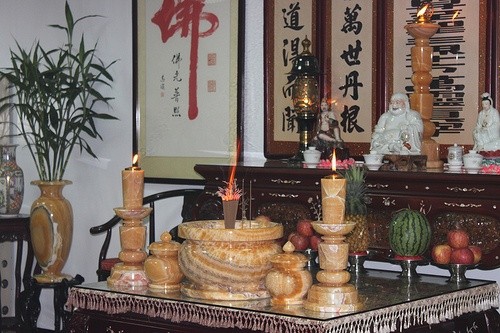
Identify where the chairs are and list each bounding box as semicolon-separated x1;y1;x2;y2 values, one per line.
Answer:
90;188;204;281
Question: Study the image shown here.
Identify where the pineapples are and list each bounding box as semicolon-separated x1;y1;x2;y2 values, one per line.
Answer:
335;165;369;252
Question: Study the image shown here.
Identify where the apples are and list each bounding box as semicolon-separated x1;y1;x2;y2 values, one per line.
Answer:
431;229;482;265
288;218;323;250
254;214;271;222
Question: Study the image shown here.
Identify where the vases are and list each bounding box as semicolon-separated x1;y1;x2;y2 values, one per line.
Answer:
0;144;24;215
30;180;73;283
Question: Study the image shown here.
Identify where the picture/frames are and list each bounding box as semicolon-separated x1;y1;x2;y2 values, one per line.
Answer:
263;0;496;160
132;0;246;186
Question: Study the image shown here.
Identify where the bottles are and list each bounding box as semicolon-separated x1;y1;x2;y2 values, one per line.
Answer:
144;231;186;293
0;143;24;215
265;241;312;308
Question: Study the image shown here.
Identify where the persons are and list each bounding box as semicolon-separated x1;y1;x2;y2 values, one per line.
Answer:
319;98;343;142
372;93;422;154
474;93;500;150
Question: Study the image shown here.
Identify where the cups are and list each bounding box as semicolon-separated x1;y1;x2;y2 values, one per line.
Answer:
362;151;384;170
446;143;464;168
461;150;483;174
300;146;322;168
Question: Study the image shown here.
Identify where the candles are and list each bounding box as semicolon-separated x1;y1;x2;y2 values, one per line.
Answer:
122;154;144;210
321;148;346;224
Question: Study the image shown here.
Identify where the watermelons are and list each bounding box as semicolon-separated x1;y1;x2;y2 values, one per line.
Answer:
388;208;432;256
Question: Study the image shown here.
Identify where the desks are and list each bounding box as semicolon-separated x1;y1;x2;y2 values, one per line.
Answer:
0;217;61;333
61;265;500;333
194;164;500;281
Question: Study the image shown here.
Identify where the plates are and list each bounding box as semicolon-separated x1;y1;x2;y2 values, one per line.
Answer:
0;214;20;219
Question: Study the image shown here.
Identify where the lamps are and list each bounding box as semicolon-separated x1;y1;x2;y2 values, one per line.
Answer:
289;35;321;161
